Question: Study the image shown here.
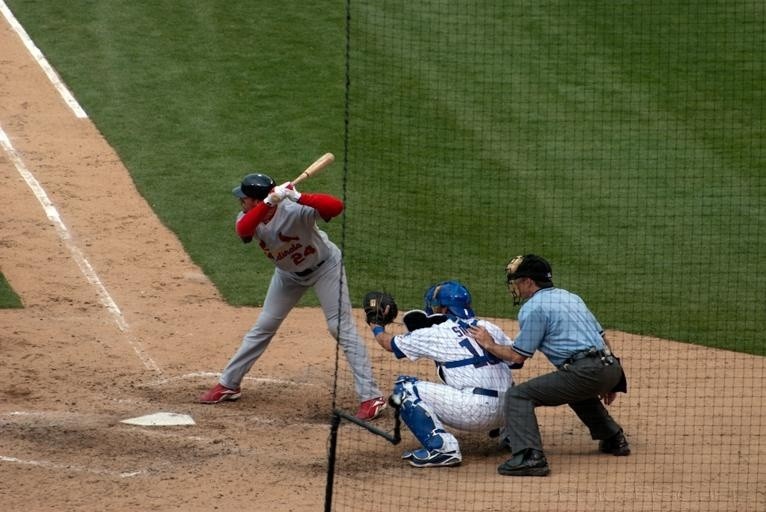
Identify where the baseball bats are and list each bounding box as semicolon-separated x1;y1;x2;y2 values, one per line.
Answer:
271;153;336;203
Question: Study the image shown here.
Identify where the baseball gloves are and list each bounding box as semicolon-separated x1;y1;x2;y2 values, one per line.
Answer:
363;291;398;327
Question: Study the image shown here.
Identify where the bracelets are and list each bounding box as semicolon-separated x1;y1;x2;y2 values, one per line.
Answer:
372;326;385;337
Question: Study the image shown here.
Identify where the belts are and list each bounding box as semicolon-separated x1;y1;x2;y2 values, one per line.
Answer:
294;260;325;277
569;350;604;359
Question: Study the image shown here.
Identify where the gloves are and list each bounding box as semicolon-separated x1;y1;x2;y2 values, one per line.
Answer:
263;181;301;208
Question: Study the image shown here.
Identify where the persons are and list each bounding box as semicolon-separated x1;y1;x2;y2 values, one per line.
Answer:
466;254;631;477
193;172;388;423
362;280;525;469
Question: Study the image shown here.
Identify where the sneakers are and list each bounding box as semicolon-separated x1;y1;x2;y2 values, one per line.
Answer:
198;383;241;403
599;430;630;456
357;397;386;421
408;450;461;467
498;449;549;476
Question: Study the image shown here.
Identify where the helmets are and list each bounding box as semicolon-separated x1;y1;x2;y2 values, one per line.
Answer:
424;281;474;319
231;173;276;200
505;255;553;307
